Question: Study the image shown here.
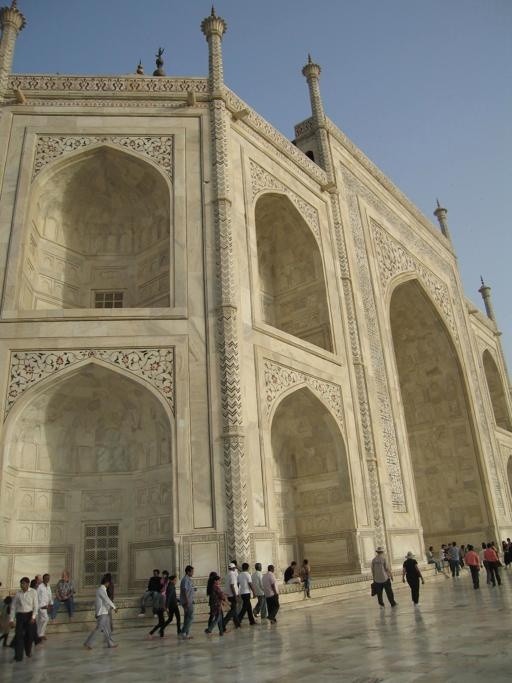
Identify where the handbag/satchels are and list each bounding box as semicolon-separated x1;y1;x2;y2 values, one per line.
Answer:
371;583;378;595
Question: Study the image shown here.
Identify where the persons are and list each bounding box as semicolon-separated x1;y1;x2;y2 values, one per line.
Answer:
301;559;311;600
1;561;279;663
427;538;512;588
284;562;301;584
402;552;424;605
371;547;398;607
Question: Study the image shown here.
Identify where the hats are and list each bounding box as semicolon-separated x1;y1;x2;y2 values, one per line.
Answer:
406;552;416;558
228;563;236;569
376;547;385;552
